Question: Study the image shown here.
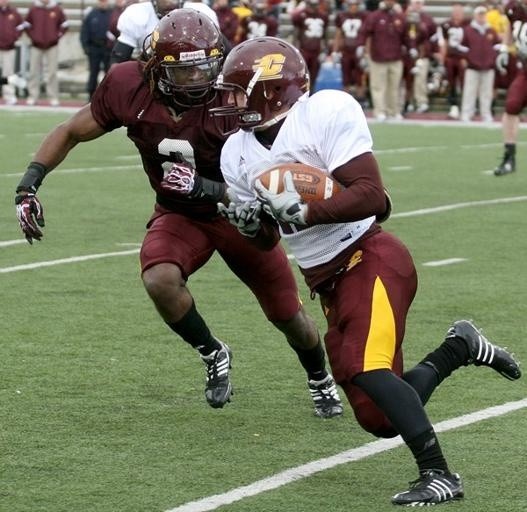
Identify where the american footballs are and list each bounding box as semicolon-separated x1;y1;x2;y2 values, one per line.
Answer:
254;162;344;204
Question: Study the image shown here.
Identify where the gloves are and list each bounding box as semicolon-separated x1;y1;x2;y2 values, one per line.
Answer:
253;171;308;226
161;151;203;199
16;195;44;244
217;188;262;238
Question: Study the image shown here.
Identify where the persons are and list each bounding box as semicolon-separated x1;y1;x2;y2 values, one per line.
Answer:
1;1;23;106
23;2;68;106
206;38;521;505
79;1;527;120
493;58;527;175
14;10;343;419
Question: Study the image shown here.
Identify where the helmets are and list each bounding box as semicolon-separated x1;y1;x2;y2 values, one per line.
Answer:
151;0;183;19
208;37;311;136
137;8;225;108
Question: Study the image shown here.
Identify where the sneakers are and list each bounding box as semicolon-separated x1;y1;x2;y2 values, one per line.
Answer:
306;367;343;418
445;319;521;381
495;158;516;175
391;468;464;507
199;338;233;408
